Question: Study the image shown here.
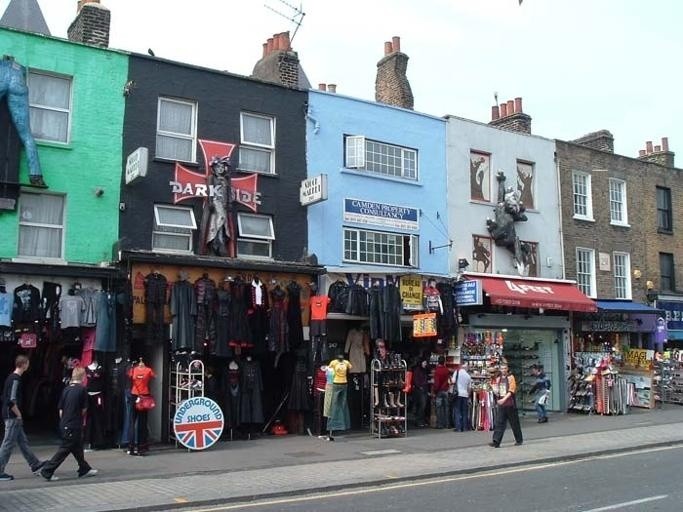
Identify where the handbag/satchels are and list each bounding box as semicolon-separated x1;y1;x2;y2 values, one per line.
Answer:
18;331;37;349
134;393;156;412
447;381;458;402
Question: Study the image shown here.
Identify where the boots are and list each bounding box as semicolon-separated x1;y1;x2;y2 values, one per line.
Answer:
373;351;405;408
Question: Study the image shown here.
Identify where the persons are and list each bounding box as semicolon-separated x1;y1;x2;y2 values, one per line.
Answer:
240;357;264;423
488;363;523;448
451;365;472;432
85;362;113;433
413;358;431;427
433;356;450;429
127;362;156;455
39;367;98;481
222;361;242;428
313;365;327;433
198;158;238;258
487;170;531;275
345;328;370;374
0;354;49;480
530;367;551;423
323;365;334;418
326;356;352;431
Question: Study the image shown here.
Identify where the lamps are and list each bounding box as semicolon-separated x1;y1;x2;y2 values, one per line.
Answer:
459;258;469;268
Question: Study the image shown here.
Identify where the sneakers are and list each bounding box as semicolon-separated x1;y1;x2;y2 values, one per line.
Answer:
29;458;48;474
512;440;523;447
536;416;548;423
69;367;85;384
78;468;98;478
0;473;13;482
36;470;59;482
486;440;500;448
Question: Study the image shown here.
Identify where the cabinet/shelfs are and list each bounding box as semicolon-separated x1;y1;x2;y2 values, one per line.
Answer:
520;334;553;411
371;358;408;439
504;334;523;410
168;359;205;452
